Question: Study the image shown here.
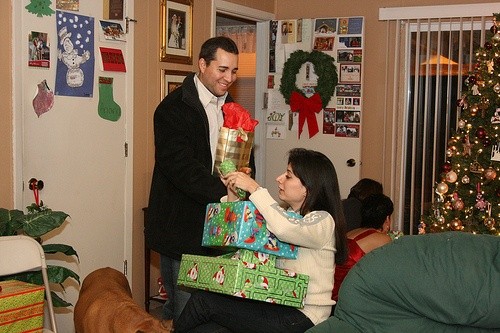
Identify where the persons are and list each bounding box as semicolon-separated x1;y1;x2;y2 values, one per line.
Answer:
30;39;43;60
353;114;360;122
330;178;394;317
323;39;330;51
336;125;356;136
173;148;348;332
345;53;352;61
324;112;334;123
339;87;345;94
168;14;184;48
351;39;358;47
146;37;256;326
343;113;350;122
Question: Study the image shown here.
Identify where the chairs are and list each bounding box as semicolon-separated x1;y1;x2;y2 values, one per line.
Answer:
0;235;57;333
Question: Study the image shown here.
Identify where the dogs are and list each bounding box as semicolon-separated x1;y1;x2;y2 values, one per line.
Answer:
73;267;175;333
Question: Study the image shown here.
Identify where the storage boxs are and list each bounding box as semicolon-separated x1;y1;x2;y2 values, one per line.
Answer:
177;157;311;309
0;279;46;333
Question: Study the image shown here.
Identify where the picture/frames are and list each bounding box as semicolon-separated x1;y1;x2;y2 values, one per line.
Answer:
160;68;198;104
159;0;194;66
